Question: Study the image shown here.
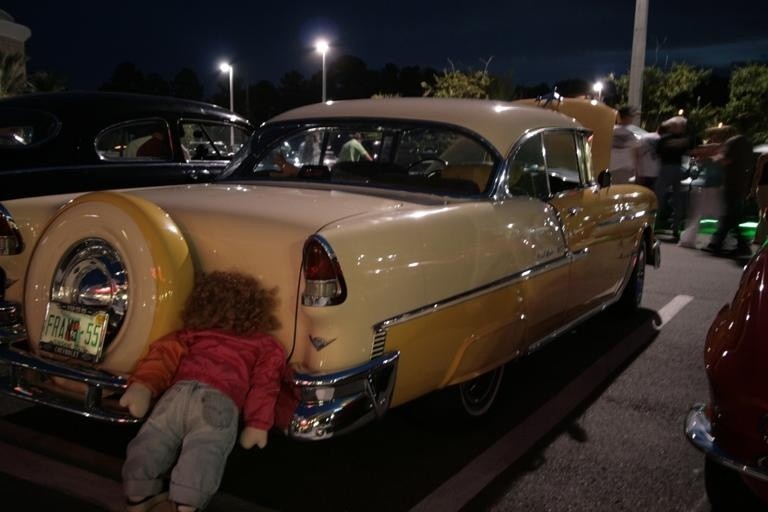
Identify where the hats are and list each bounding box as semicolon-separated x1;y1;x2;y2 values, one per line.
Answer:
619;106;644;117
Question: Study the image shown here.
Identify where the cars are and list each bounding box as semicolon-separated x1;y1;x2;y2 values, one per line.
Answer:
0;91;305;207
683;150;768;512
1;92;661;442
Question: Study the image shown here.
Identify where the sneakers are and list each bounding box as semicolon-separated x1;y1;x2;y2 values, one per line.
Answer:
700;245;722;255
729;247;752;257
671;222;679;236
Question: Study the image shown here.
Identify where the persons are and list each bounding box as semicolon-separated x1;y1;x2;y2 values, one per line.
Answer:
690;115;756;258
649;94;700;242
636;115;667;195
749;152;767;245
333;130;374;162
603;103;643;185
116;268;288;512
